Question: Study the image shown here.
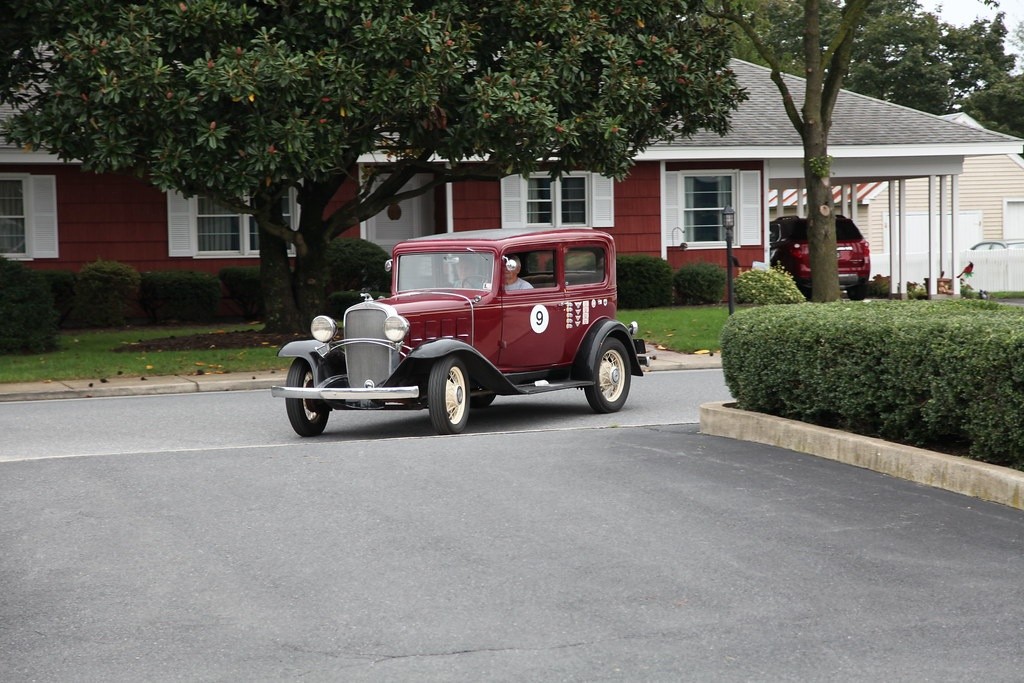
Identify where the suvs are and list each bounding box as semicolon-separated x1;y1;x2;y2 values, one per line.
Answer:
770;215;871;304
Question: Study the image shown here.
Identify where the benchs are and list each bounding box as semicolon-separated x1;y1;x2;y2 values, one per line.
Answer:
521;274;598;288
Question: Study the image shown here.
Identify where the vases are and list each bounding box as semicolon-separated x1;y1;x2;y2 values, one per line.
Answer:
924;277;951;293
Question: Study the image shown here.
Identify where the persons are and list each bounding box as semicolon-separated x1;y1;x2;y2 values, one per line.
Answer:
455;257;476;289
502;255;534;289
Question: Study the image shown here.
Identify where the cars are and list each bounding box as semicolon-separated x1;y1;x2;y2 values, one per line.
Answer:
271;228;650;435
971;238;1024;253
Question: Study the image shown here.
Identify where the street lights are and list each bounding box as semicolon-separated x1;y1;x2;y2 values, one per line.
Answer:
722;205;737;315
